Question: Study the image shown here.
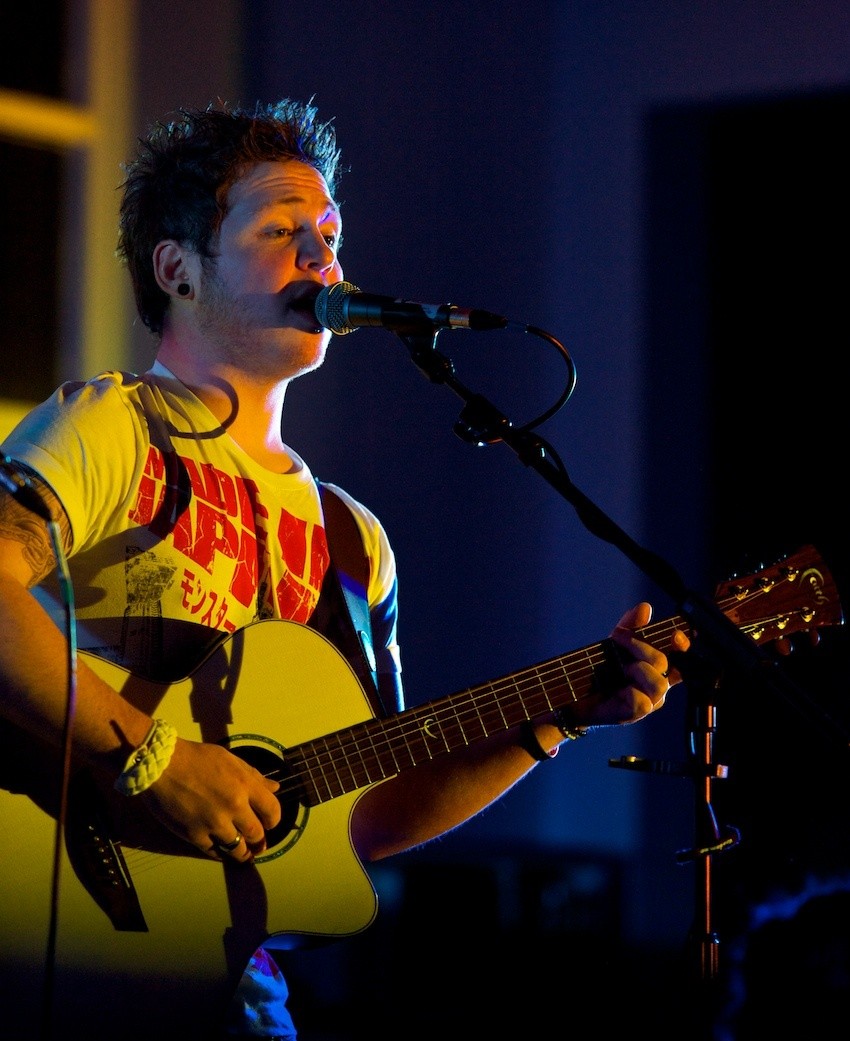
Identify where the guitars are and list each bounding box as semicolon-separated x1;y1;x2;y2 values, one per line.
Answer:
0;547;850;1041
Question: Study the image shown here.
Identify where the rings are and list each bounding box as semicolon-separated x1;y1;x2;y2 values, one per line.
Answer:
216;831;241;852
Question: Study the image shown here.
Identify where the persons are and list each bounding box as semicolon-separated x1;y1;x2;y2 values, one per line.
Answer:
0;98;693;1041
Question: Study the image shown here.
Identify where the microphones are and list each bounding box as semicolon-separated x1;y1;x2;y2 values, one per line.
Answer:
315;282;510;335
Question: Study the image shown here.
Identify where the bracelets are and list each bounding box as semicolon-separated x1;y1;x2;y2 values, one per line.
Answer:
554;708;587;741
113;718;178;796
518;722;559;761
